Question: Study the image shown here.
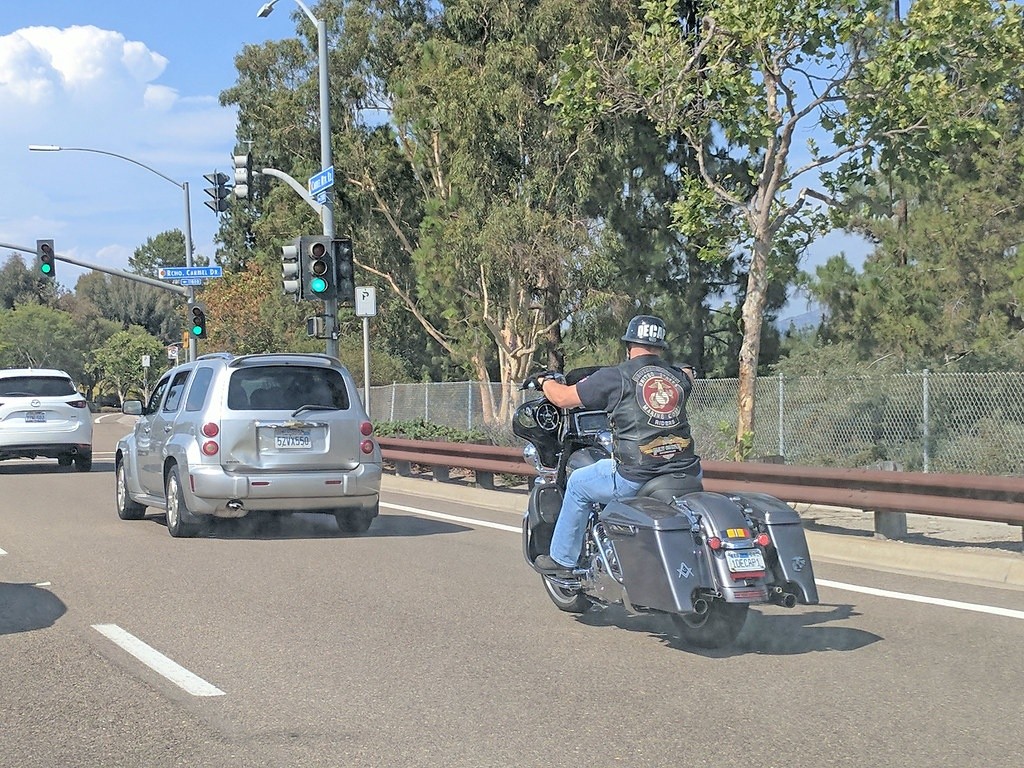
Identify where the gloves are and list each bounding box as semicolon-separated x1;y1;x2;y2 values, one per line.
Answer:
523;372;555;391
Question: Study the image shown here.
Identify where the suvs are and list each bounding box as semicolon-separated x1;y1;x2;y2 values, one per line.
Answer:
0;367;93;472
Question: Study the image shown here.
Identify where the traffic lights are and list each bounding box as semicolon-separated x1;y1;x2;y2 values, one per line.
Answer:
281;235;301;304
308;317;323;338
37;239;55;277
188;302;206;339
331;238;355;300
301;235;333;301
231;152;252;203
217;172;232;211
202;169;217;218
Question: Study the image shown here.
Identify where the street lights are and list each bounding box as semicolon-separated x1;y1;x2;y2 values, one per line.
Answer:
257;0;340;359
30;145;198;363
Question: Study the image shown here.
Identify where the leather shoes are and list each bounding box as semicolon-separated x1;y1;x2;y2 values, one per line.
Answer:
534;555;577;578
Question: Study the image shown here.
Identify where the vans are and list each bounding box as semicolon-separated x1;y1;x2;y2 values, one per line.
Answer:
114;353;383;538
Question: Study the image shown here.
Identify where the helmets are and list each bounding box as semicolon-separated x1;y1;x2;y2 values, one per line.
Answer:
621;315;667;347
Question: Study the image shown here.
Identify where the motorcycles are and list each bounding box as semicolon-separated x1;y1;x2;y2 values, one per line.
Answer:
511;360;819;648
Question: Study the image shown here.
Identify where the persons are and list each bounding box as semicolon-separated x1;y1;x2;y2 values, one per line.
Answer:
530;315;703;580
